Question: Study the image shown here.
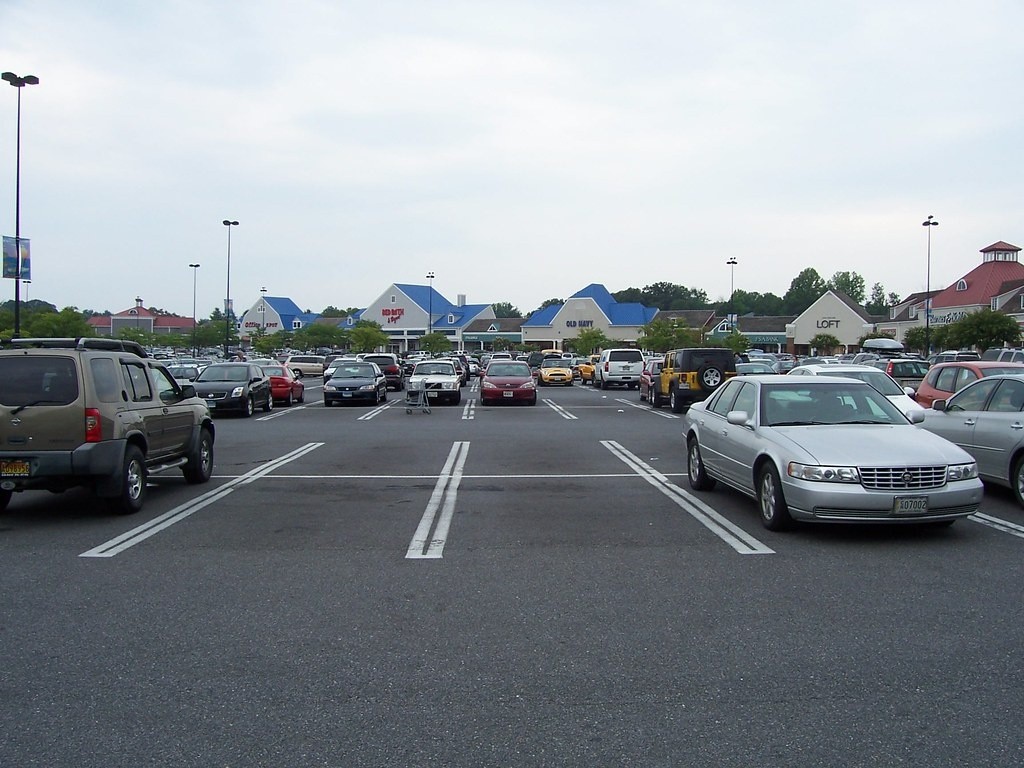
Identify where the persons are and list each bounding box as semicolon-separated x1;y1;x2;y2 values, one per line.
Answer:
237;348;243;361
269;350;278;360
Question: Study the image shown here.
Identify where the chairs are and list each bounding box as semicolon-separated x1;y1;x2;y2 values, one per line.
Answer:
817;394;849;419
558;362;564;366
421;366;431;373
546;362;552;366
441;366;450;373
964;375;976;385
516;367;524;373
1009;388;1024;409
905;367;913;374
941;374;954;388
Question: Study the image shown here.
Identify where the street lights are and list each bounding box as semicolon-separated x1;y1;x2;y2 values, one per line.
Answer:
189;264;200;355
426;271;435;333
726;257;738;333
223;220;239;356
260;286;267;336
923;215;939;346
3;70;38;338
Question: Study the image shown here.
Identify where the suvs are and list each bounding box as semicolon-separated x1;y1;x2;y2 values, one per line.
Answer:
649;348;737;413
448;354;471;381
0;336;215;512
285;355;326;379
362;353;406;391
592;349;646;390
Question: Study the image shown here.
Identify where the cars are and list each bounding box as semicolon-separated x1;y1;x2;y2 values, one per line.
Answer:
681;347;1023;529
143;344;434;416
639;360;664;404
408;360;463;405
434;349;667;387
481;361;537;405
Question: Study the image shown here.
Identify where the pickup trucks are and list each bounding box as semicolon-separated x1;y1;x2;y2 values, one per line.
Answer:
578;355;600;385
